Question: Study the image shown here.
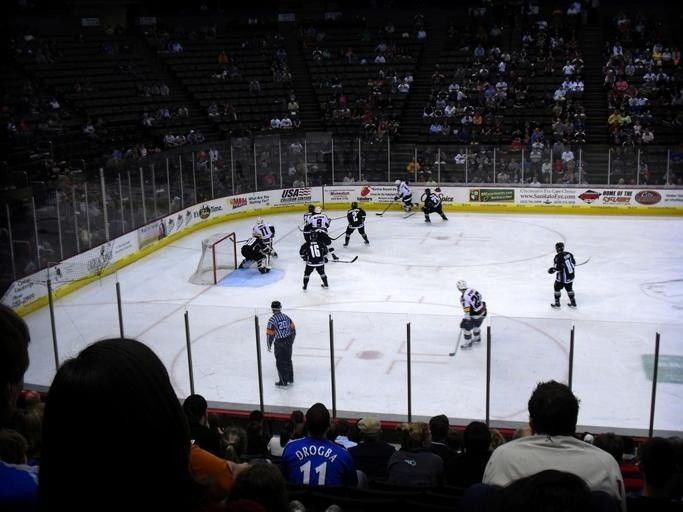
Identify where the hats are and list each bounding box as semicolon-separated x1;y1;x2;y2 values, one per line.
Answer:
357;416;383;434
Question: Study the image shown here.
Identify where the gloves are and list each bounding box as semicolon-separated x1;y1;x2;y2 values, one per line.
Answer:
460;319;474;331
548;267;555;275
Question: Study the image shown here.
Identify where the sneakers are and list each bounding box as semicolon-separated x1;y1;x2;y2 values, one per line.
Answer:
551;302;560;307
461;343;472;349
567;302;576;306
471;338;480;343
275;381;287;386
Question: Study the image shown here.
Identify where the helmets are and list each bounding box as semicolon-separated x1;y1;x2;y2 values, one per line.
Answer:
394;180;401;187
256;216;264;224
308;204;322;214
309;231;318;241
457;279;469;290
556;243;564;253
351;202;357;209
271;301;282;311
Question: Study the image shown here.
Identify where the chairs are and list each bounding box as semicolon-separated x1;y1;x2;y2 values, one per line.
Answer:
4;4;224;262
227;1;683;197
0;377;681;509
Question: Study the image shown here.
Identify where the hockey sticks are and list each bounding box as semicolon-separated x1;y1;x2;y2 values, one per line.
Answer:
449;328;463;356
376;199;395;215
329;232;345;240
230;237;247;243
328;256;358;264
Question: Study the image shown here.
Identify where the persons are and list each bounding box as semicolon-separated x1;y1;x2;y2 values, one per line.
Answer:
0;243;683;512
2;2;681;288
